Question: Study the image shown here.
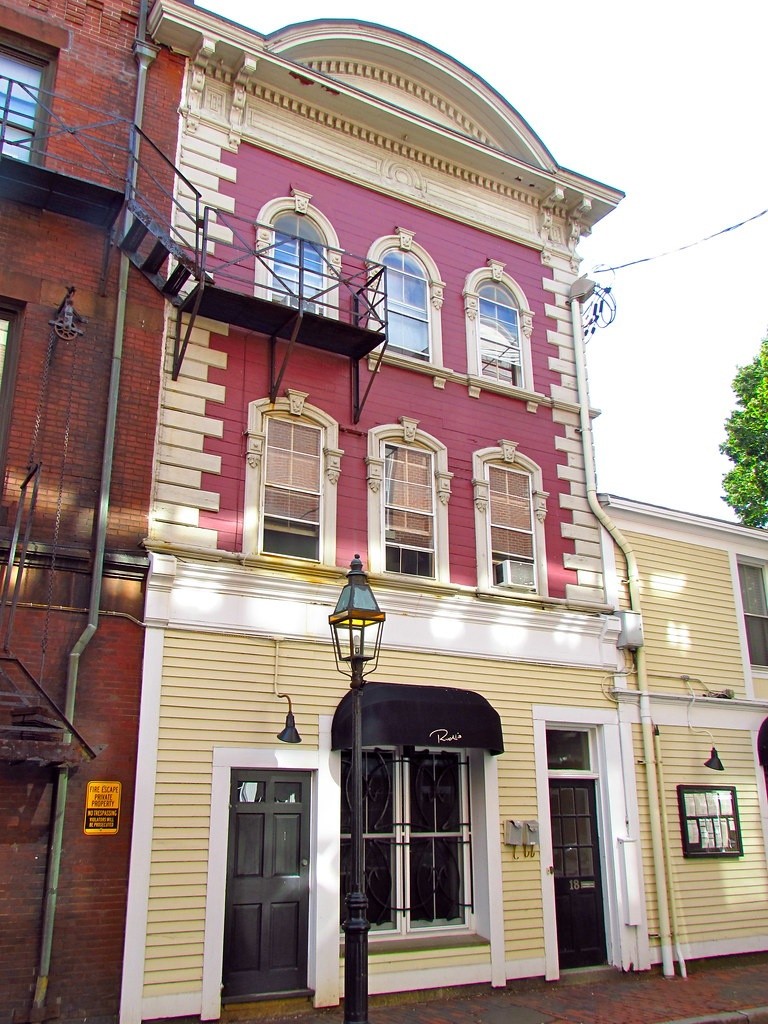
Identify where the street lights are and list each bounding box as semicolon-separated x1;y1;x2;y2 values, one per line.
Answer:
327;553;386;1023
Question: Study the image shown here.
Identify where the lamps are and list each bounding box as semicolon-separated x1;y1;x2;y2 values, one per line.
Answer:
274;635;302;744
684;676;725;771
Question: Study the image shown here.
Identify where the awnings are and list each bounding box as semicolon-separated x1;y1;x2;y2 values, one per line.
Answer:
331;682;504;758
